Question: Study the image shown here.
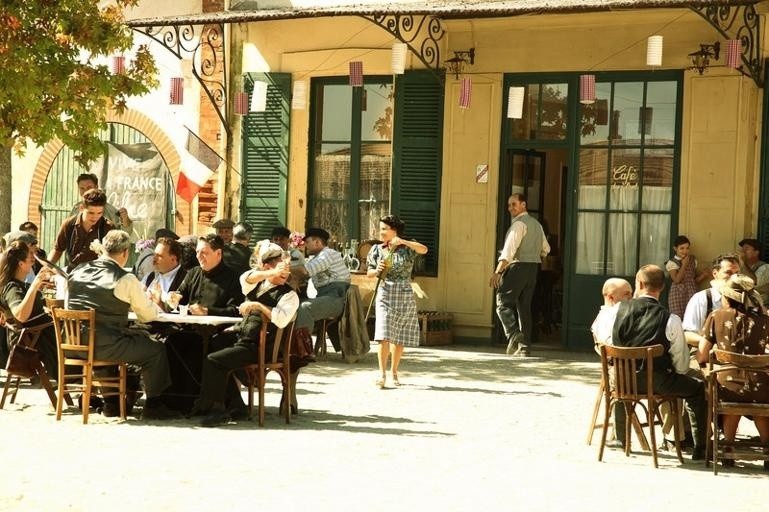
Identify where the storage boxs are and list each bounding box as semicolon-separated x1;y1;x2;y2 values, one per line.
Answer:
418;310;454;347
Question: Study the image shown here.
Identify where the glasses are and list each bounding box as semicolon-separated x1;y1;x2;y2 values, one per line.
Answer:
23;258;36;266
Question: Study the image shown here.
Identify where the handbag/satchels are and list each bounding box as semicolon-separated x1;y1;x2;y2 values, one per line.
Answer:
289;326;314;374
5;327;41;379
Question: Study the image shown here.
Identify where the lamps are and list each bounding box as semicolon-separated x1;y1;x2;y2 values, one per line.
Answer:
349;61;363;87
392;43;407;75
687;41;720;76
113;56;125;75
233;92;249;116
444;47;475;80
169;78;183;105
579;74;595;104
507;86;525;119
458;79;472;109
646;35;663;66
724;39;742;68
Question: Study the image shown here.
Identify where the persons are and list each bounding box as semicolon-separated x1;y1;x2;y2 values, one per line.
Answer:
490;192;551;358
592;237;769;469
366;216;428;388
47;189;114;274
0;219;350;421
69;175;133;236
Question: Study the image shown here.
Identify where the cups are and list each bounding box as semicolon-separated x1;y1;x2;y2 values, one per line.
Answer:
169;291;182;314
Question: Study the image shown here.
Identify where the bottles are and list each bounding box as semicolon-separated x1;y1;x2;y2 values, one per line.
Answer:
249;256;260;277
281;251;293;274
379;244;398;279
342;240;362;270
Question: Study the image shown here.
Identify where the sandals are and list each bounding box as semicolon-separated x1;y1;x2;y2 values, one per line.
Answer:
376;378;402;389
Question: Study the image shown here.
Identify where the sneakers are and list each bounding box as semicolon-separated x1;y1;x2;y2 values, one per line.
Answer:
722;445;736;467
103;391;137;417
77;394;104;413
691;446;718;460
143;402;182;418
198;405;248;427
505;331;531;357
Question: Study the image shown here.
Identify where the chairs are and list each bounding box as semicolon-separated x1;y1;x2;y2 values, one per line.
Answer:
703;349;769;475
597;342;684;469
51;305;128;424
0;314;74;412
588;335;623;446
313;284;359;362
225;311;297;427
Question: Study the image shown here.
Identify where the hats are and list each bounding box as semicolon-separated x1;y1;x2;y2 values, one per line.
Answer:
4;231;35;244
213;219;252;237
155;228;180;240
737;237;761;251
709;273;763;309
301;227;330;242
272;227;291;238
